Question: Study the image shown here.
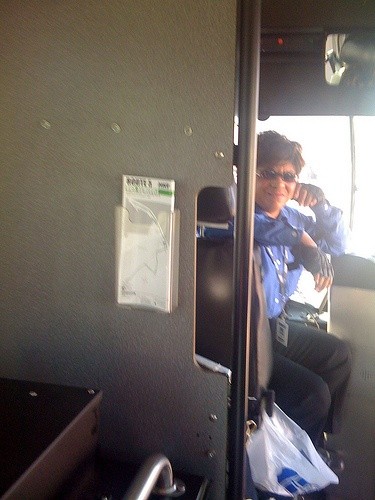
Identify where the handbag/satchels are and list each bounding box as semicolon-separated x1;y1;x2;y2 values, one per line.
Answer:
247;397;341;496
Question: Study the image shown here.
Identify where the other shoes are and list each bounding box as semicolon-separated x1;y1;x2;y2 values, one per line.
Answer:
317;444;344;474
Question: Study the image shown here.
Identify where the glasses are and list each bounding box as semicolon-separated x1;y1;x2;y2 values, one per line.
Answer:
255;168;298;182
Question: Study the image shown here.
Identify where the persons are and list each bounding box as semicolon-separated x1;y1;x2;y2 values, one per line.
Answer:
193;129;353;475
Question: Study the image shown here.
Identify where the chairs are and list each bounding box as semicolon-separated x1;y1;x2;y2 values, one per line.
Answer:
195;181;276;418
